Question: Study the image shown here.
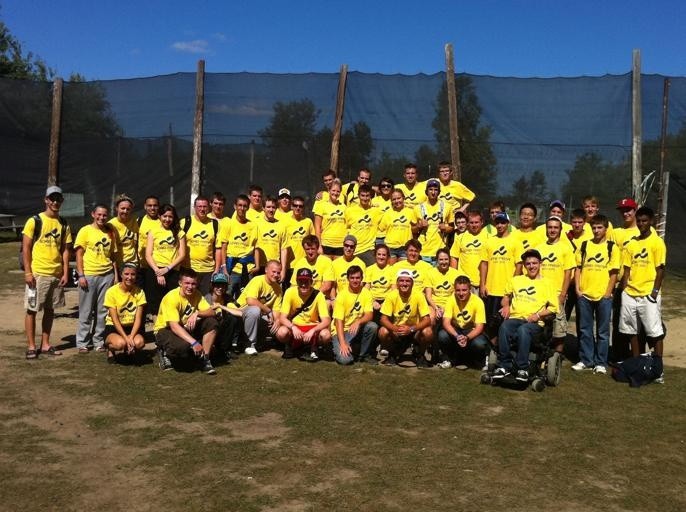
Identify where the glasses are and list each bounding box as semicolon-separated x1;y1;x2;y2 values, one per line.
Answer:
380;184;392;188
293;204;304;208
48;195;64;202
278;196;292;199
343;242;356;247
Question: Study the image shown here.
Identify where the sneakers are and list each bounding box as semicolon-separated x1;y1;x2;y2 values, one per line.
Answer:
158;349;172;370
593;365;607;375
363;356;378;364
283;344;294;357
107;353;116;364
300;352;317;362
515;370;528;381
493;368;510;378
244;347;257;355
572;362;592;371
196;359;215;373
438;360;452;369
654;373;664;383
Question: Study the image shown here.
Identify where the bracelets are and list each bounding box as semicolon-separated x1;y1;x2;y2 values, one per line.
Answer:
25;273;32;276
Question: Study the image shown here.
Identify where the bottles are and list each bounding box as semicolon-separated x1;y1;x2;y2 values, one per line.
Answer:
27;287;38;309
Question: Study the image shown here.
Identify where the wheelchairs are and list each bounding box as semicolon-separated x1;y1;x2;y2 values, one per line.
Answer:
488;297;567;391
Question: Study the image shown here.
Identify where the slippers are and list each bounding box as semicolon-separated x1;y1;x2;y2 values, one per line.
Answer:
27;350;37;359
79;348;88;354
94;347;106;353
40;347;62;356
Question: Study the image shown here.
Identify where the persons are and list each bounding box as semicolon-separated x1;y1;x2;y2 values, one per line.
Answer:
70;159;667;386
21;185;72;360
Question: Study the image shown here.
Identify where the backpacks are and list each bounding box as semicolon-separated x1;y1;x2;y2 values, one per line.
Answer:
19;214;66;270
611;350;662;388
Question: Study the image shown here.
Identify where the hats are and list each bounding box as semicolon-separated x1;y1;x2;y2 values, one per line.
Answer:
396;269;414;280
521;249;541;259
549;200;565;210
547;215;562;224
455;211;467;216
495;212;510;221
46;186;62;196
277;188;292;196
297;268;312;280
210;273;228;283
427;180;440;187
344;235;357;245
615;198;638;209
